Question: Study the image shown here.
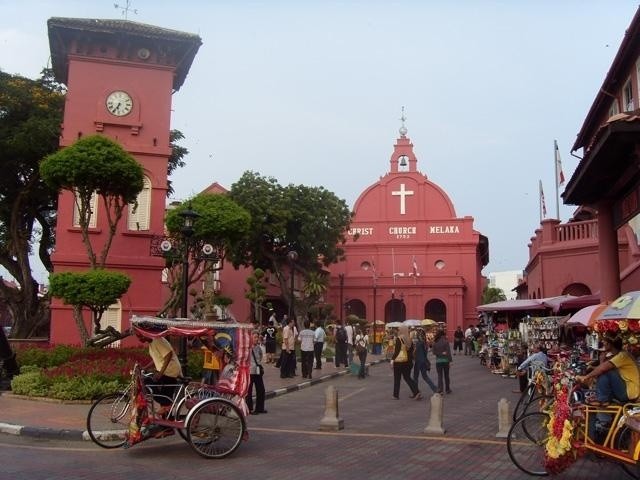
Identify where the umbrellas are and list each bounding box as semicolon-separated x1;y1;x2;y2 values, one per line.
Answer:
566;297;614;328
595;290;640;321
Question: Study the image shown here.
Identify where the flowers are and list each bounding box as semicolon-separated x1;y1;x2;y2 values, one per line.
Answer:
541;339;595;474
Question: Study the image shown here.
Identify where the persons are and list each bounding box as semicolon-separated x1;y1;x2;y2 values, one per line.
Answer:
253;311;519;382
512;341;529;393
517;344;548;393
431;330;453;396
245;330;268;415
388;326;422;400
408;329;438;398
575;332;640;406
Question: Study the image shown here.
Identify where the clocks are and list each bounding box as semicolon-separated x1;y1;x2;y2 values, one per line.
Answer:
94;85;144;135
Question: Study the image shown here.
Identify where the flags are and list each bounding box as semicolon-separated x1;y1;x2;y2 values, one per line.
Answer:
539;182;547;218
412;260;421;279
556;145;566;187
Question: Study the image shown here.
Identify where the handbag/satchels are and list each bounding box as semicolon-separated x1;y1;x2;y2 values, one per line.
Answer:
256;363;264;376
436;355;449;368
425;356;431;371
352;335;356;346
392;343;409;364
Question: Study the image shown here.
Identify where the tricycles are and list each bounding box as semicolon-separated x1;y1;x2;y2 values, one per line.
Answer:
504;291;639;479
85;312;256;458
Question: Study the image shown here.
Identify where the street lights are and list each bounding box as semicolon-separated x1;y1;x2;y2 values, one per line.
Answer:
371;275;380;353
391;289;396;322
399;292;405;322
178;205;202;379
286;250;299;321
338;271;345;323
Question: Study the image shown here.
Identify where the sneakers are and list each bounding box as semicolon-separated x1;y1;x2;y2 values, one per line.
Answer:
439;391;444;396
334;366;350;369
255;409;268;413
155;429;175;439
156;404;175;416
390;395;399;400
248;410;259;415
408;391;424;400
445;388;452;394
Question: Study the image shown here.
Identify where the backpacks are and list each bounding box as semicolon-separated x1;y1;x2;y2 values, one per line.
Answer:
357;334;367;354
336;325;348;345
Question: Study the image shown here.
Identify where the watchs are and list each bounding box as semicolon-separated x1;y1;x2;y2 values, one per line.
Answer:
134;330;184;439
